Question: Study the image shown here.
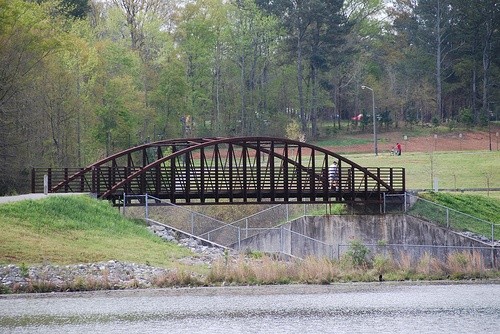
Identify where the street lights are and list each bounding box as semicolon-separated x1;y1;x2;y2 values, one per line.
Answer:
361;85;377;156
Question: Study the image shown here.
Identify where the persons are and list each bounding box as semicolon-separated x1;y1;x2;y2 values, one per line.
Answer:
351;115;357;125
396;143;402;156
327;160;340;190
389;146;396;156
